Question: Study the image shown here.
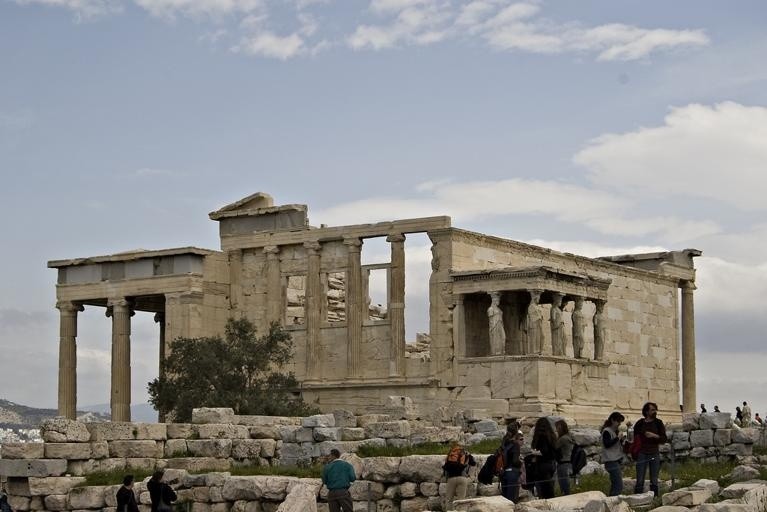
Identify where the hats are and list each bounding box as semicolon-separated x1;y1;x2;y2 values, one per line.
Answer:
507;421;518;434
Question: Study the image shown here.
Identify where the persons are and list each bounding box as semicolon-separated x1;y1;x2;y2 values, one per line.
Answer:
599;412;631;496
755;413;761;423
442;451;476;479
571;301;588;359
713;405;719;411
740;401;751;428
734;407;743;428
530;417;557;499
550;297;568;356
528;295;546;354
499;421;523;504
146;471;177;511
114;474;139;511
570;439;586;476
487;297;506;356
591;304;606;361
0;488;13;511
632;402;667;497
515;433;526;485
554;420;574;495
321;448;356;511
476;447;498;483
700;403;706;413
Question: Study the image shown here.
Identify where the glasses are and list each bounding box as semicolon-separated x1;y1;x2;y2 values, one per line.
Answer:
520;438;523;441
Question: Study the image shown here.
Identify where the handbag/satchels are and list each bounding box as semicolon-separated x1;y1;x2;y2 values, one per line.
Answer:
522;454;543;490
495;446;504;473
122;504;128;511
572;444;587;473
157;500;172;511
442;462;466;475
